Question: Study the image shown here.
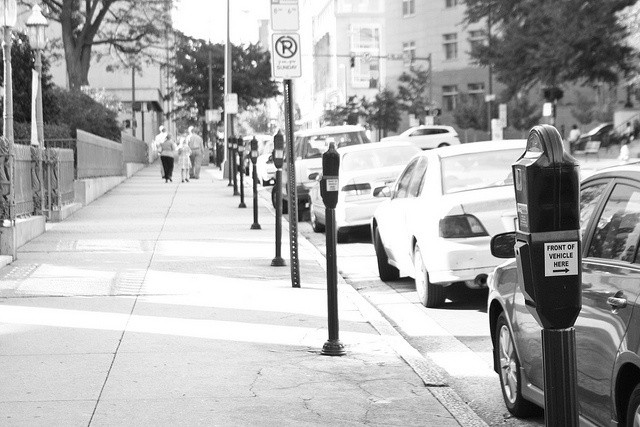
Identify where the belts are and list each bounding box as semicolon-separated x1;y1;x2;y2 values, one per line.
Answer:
191;148;200;149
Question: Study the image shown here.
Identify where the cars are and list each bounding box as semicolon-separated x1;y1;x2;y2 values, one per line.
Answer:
373;139;527;307
569;123;614;149
238;136;269;176
272;127;371;222
256;138;281;187
490;151;640;426
391;124;462;149
311;137;423;245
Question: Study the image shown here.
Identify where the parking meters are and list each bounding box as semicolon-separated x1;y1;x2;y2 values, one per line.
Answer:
512;124;583;427
321;141;346;357
248;136;261;230
232;136;239;196
268;134;289;266
215;136;225;172
228;136;234;186
238;137;246;208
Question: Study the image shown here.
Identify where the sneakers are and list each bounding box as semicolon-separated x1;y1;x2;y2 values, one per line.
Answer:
182;180;184;183
185;177;189;182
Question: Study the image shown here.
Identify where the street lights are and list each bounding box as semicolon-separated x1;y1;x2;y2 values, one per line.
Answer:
1;0;20;142
27;4;50;147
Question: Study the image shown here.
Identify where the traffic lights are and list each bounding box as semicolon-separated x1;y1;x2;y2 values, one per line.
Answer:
350;55;356;67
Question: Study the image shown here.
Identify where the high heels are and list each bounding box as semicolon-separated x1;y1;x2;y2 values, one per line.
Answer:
165;177;168;182
169;177;172;182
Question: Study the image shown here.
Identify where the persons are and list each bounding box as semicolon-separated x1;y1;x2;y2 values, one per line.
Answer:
178;137;192;183
186;126;204;179
156;125;168;178
160;134;175;183
